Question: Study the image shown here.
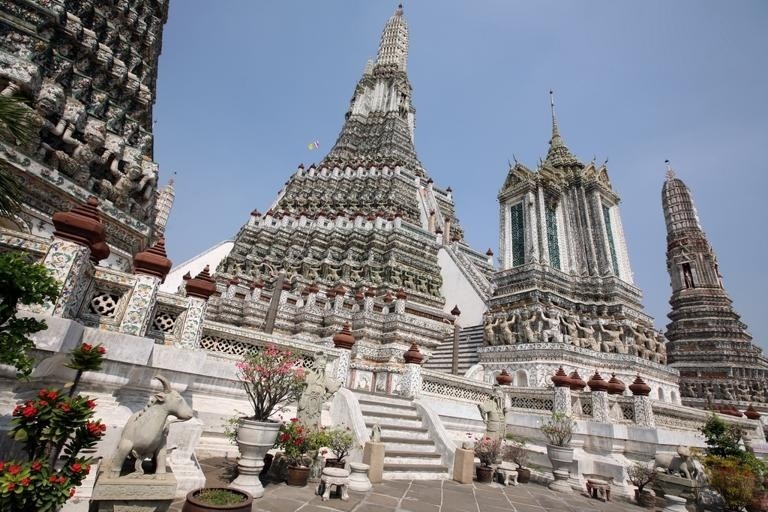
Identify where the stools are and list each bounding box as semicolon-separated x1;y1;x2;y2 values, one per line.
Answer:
314;467;351;502
496;461;519;486
586;477;611;504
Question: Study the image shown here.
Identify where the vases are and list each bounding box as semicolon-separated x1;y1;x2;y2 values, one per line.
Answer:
633;488;649;502
287;464;310;487
475;465;493;483
515;468;529;484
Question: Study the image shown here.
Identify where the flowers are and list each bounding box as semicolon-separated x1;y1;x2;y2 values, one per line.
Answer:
231;339;309;422
504;441;530;469
273;413;309;467
464;432;503;469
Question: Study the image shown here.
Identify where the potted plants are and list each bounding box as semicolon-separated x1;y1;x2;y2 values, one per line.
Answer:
180;487;254;512
319;423;351;469
537;408;579;493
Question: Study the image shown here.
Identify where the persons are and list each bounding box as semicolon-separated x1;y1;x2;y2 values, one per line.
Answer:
477;383;509;464
296;351;342;433
